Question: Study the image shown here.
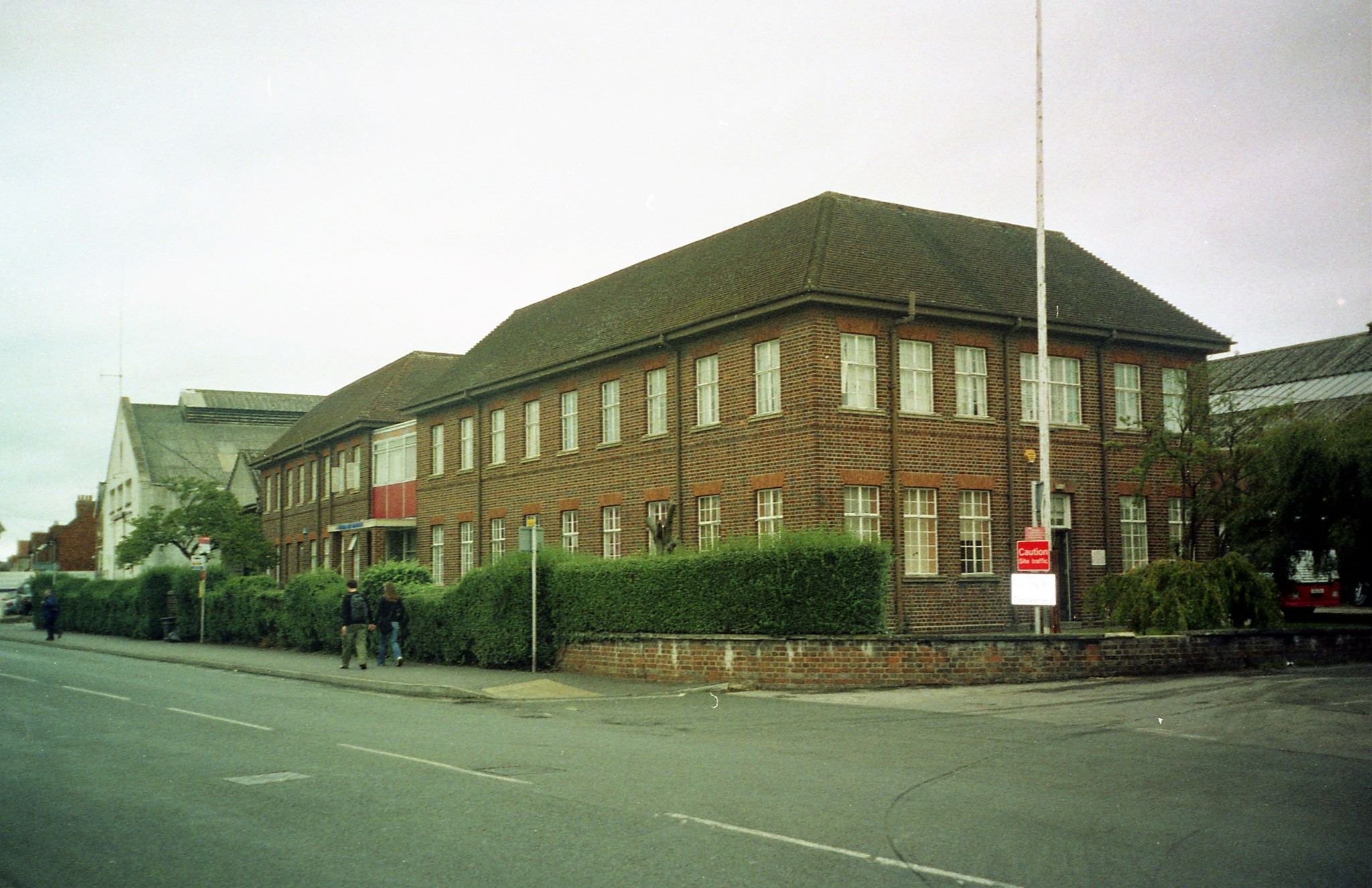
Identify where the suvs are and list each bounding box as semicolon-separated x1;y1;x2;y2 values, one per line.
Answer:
1213;510;1340;621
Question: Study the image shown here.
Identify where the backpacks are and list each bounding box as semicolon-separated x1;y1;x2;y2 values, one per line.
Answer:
346;593;368;621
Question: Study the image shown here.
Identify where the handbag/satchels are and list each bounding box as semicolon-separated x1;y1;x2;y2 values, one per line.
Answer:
378;619;394;635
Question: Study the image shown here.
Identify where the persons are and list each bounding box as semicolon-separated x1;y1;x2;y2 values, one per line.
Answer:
368;582;409;668
40;589;63;641
339;580;377;669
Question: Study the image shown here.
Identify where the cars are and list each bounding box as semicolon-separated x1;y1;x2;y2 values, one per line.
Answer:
0;579;38;616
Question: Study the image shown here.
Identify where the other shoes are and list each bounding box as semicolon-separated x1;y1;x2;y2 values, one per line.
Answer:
58;630;63;639
396;657;403;667
339;664;349;668
46;637;54;641
360;663;366;669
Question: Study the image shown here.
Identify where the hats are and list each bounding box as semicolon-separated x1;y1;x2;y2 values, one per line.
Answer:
346;580;357;586
